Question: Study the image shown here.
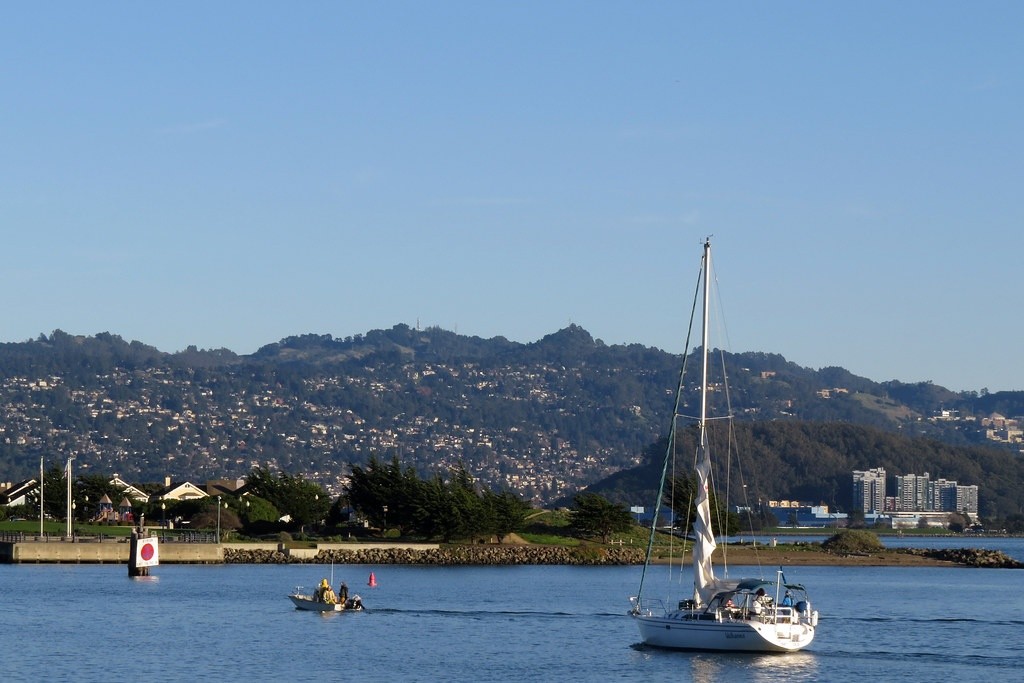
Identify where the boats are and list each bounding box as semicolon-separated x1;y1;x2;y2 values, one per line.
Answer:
287;586;362;612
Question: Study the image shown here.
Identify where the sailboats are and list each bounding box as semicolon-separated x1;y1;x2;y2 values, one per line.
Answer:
627;234;819;652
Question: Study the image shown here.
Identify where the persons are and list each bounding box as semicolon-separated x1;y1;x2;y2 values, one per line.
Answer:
783;589;795;615
324;586;338;605
339;582;348;605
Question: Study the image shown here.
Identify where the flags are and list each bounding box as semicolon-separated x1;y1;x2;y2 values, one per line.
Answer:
136;537;159;568
